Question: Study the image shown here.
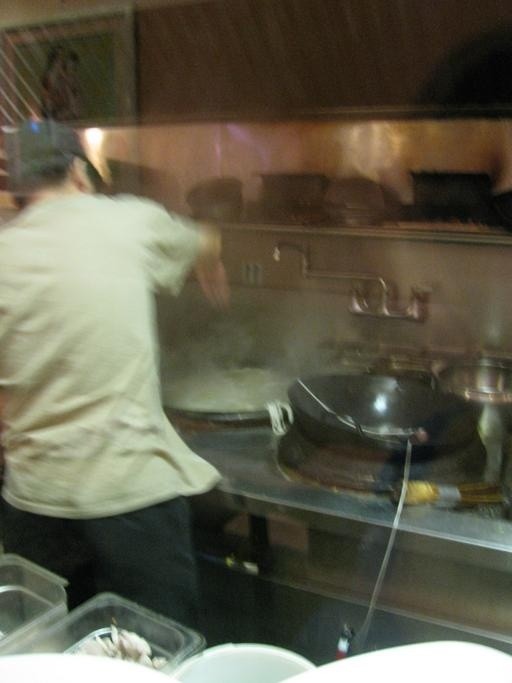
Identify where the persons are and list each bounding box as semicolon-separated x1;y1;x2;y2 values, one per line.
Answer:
0;117;225;640
56;49;87;117
40;43;65;116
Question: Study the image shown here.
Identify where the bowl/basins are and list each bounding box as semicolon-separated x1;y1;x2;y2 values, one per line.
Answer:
174;642;315;683
431;356;512;418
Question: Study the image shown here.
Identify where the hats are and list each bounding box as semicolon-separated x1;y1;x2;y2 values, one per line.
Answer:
6;119;109;188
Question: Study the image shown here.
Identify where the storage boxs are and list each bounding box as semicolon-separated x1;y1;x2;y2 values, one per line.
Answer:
0;554;69;646
1;591;207;674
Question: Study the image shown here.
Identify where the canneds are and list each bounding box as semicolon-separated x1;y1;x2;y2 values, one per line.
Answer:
392;481;461;509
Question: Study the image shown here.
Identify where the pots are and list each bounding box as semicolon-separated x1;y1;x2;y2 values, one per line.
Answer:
287;374;464;462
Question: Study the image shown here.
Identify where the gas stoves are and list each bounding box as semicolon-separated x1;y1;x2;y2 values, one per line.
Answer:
160;425;512;665
